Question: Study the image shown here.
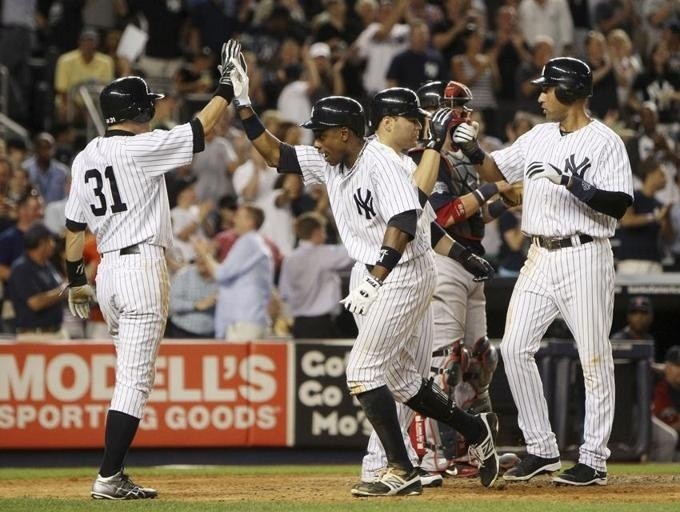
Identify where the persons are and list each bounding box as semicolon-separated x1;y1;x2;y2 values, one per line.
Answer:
0;0;679;342
358;84;496;488
611;296;680;462
451;56;635;489
62;39;243;504
226;54;502;498
406;78;522;477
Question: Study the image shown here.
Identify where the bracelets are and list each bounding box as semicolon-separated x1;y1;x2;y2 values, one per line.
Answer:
473;182;498;206
64;255;89;287
374;247;401;272
566;177;595;203
241;112;266;140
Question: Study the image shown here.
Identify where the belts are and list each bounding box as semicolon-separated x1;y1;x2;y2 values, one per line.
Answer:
120;245;142;256
529;233;593;251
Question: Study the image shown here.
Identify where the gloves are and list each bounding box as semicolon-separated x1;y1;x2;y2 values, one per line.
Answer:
526;160;569;189
427;106;457;150
460;246;498;283
339;276;381;314
68;285;98;319
218;39;253;108
453;122;480;157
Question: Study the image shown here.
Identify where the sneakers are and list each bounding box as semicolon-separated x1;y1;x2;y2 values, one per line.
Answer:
90;466;157;500
350;412;608;495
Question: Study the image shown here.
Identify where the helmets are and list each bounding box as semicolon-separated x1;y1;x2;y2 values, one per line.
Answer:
369;85;432;122
419;77;470;126
531;58;593;96
300;96;364;134
100;76;165;125
